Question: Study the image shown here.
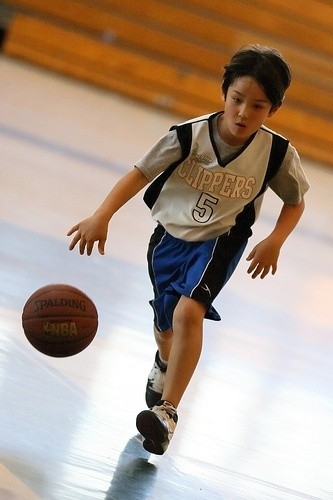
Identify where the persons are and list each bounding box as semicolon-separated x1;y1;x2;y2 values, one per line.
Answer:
67;47;311;456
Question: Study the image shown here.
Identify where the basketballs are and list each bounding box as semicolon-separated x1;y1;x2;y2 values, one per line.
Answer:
22;283;98;358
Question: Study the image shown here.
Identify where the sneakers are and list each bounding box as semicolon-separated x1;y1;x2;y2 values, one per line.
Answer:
136;400;179;455
145;349;169;411
